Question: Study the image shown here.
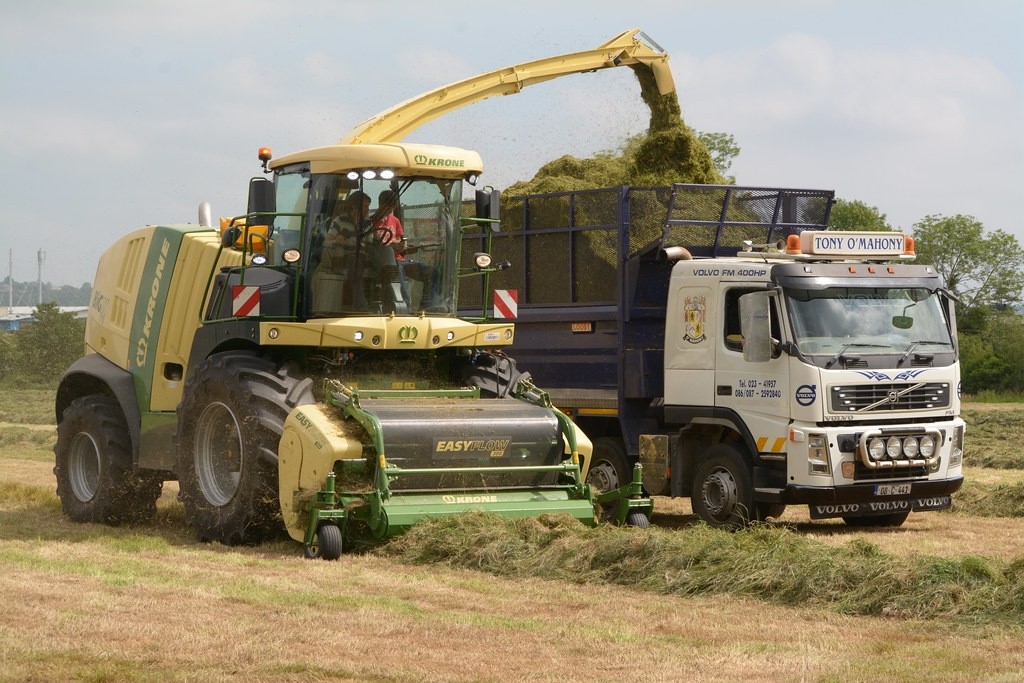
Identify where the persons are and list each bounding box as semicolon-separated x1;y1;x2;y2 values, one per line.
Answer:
329;190;448;318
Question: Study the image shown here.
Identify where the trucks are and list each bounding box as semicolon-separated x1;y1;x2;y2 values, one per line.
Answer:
364;182;967;532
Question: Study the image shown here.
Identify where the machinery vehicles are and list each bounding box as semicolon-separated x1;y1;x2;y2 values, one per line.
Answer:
53;27;681;561
